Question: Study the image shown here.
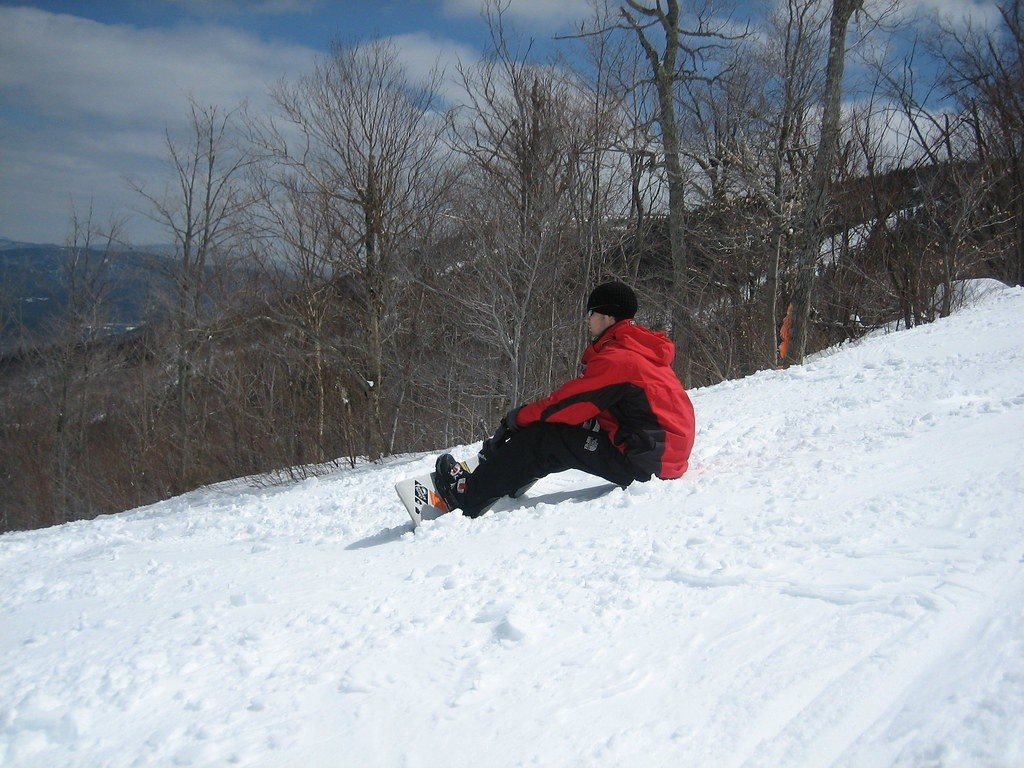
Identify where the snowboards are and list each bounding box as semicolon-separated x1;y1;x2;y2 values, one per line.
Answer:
393;453;482;529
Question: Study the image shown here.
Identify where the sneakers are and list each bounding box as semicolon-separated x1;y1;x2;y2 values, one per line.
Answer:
436;454;470;510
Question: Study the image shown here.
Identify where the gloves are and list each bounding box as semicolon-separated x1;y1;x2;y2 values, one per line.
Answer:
491;418;512;451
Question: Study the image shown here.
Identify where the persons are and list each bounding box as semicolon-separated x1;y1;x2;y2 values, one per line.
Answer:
435;281;695;517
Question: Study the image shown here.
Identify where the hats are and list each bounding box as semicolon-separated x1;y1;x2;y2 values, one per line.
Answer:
588;282;637;318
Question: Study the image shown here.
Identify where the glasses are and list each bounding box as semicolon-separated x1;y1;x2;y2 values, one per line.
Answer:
587;304;619;317
